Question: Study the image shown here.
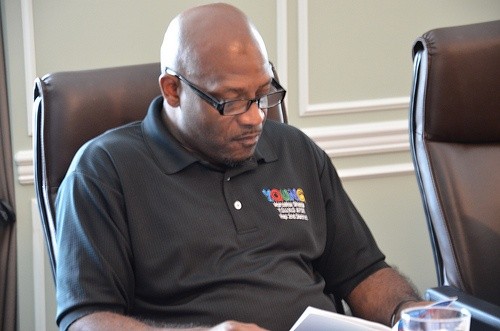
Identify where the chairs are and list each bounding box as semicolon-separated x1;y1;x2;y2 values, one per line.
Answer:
33;62;346;316
409;20;500;331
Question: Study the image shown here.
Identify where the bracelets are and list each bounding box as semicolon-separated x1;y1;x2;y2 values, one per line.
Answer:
390;298;419;328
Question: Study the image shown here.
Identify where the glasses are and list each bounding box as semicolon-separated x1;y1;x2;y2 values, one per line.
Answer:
165;61;287;115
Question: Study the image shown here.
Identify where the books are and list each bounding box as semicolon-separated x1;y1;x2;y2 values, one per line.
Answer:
289;296;461;331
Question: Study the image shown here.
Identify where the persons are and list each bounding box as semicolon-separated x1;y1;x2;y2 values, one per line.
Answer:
56;5;460;331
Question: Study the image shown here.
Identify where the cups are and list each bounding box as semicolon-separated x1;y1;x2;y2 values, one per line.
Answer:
400;306;471;331
